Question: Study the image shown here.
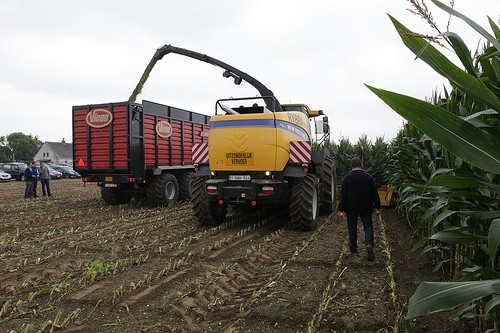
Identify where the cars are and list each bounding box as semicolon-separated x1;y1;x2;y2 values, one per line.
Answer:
47;163;81;179
0;170;12;182
35;162;63;180
0;163;40;182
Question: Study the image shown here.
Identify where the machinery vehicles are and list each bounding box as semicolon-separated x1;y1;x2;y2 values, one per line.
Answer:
154;44;395;231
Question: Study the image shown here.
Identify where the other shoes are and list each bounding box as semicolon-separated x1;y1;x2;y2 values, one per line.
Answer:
34;194;37;197
366;244;375;261
48;194;52;197
25;195;28;199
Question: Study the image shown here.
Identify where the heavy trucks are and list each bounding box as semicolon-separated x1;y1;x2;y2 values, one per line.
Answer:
72;100;212;206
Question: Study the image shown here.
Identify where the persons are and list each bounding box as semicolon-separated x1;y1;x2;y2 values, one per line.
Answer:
38;162;53;197
24;161;37;198
336;157;381;261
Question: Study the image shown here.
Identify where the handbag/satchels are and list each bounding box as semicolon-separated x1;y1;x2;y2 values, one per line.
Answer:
337;198;347;211
